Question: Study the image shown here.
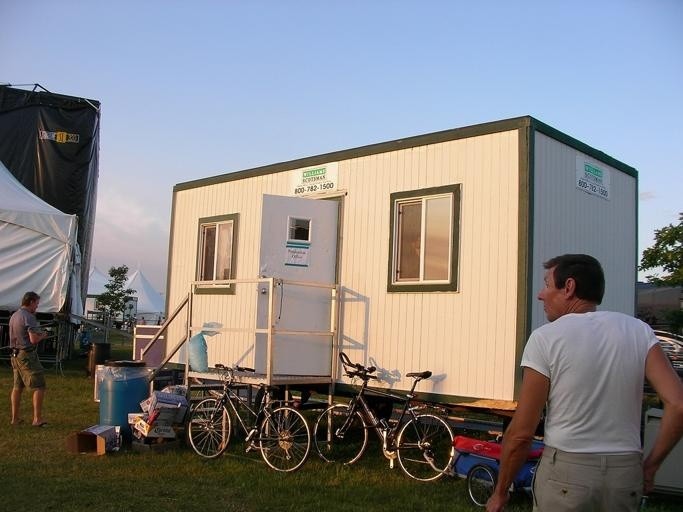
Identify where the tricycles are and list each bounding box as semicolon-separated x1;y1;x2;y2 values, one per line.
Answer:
455;432;649;511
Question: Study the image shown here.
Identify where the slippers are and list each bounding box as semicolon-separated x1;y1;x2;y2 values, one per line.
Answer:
10;419;25;425
32;422;53;428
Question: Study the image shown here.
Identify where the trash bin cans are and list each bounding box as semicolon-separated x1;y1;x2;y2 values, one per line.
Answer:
88;343;111;372
99;361;150;443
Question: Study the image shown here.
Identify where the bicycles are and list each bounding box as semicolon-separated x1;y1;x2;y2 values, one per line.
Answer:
313;350;456;483
186;363;312;472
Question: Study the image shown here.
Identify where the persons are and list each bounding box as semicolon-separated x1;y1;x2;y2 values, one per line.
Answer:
483;251;683;512
159;316;165;324
137;316;145;325
8;290;54;428
157;316;161;325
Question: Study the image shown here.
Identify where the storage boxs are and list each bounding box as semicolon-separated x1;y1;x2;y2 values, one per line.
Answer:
64;384;188;457
